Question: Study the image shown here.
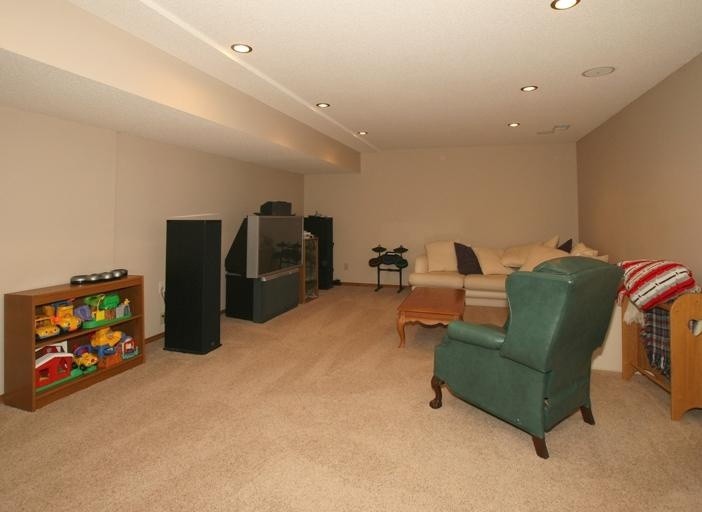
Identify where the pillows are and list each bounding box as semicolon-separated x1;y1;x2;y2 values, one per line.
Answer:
616;258;696;311
425;235;609;274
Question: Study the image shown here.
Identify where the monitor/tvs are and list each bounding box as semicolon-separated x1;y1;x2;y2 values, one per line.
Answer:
223;215;305;282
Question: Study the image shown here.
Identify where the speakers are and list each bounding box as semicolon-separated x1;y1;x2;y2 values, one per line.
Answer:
260;201;292;215
302;216;334;290
163;219;223;355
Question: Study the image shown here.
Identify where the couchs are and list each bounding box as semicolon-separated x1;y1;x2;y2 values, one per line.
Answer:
410;253;623;371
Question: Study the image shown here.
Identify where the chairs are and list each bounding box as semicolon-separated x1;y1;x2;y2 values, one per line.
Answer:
428;255;626;459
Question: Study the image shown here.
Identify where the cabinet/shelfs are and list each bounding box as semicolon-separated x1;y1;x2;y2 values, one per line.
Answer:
621;290;702;421
224;268;299;322
299;238;319;303
4;276;144;412
